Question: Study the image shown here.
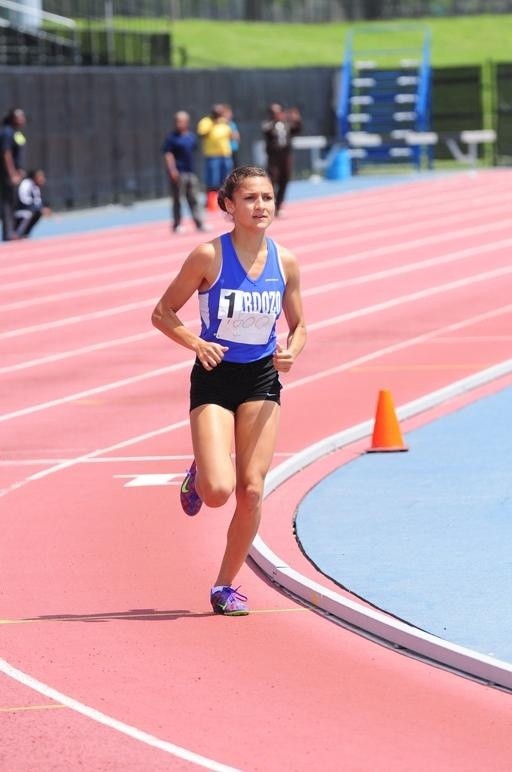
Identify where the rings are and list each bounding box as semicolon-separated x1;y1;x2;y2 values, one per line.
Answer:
206;356;211;360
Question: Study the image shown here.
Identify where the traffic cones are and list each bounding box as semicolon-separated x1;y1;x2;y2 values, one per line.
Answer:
364;389;412;457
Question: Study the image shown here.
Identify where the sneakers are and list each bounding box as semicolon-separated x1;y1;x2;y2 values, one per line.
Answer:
210;586;249;617
180;460;203;517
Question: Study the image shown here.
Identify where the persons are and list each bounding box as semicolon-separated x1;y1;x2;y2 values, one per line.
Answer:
261;104;303;216
223;104;242;171
195;104;236;214
160;111;212;234
151;164;307;617
1;105;27;241
13;167;53;240
